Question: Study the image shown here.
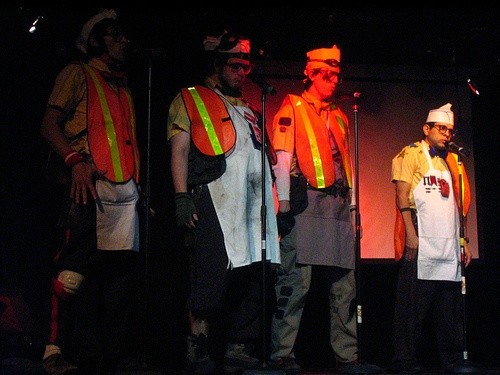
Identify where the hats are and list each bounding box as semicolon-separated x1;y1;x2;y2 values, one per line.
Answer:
306;45;341;73
75;9;117;54
425;103;455;127
204;30;251;55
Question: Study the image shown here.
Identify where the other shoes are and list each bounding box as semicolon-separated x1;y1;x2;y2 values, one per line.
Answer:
270;355;300;373
42;353;78;374
396;361;422;375
184;337;215;373
335;357;382;373
225;342;258;368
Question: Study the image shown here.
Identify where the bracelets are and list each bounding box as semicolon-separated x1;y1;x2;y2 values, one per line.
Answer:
464;237;469;243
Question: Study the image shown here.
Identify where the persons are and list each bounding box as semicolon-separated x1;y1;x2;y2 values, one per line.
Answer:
41;9;141;375
166;30;281;375
269;45;381;375
389;103;475;375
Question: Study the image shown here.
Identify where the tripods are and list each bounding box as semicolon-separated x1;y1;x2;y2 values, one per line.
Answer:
440;153;486;374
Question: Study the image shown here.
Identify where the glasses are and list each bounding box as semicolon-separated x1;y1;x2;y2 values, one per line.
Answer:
225;63;252;74
432;124;457;137
103;30;129;42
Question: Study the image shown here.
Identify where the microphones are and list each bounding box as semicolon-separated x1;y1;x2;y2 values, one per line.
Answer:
446;140;471;157
249;71;275;96
342;88;364;99
